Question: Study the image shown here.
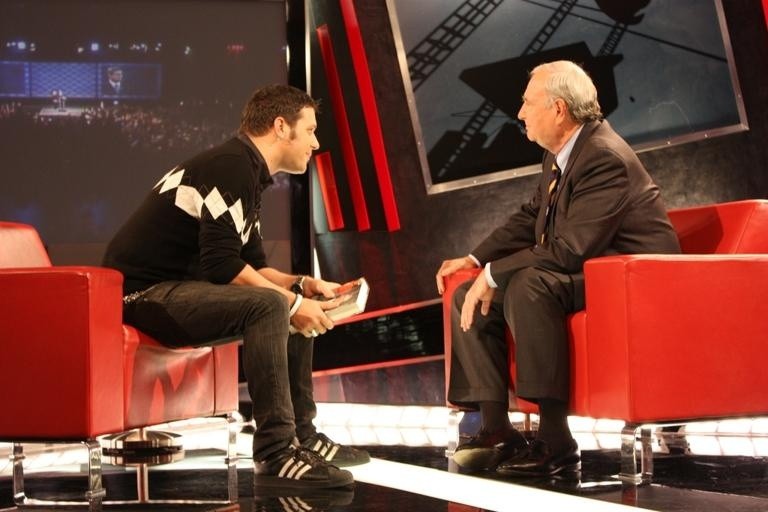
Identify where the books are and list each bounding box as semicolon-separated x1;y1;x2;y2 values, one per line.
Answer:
288;276;370;334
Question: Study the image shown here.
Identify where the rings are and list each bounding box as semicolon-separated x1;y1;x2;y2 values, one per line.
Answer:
311;331;319;339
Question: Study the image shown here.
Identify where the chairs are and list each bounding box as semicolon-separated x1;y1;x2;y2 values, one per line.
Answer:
442;200;768;487
1;222;239;509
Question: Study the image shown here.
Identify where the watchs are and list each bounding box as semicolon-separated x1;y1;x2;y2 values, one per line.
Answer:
290;272;307;296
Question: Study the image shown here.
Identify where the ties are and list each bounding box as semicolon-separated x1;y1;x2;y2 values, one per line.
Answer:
541;161;561;243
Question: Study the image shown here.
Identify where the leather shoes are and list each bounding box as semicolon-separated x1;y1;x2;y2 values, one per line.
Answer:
497;439;579;476
453;430;529;467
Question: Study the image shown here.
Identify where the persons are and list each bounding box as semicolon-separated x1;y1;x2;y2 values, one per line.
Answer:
436;61;680;476
99;65;129;95
99;82;371;488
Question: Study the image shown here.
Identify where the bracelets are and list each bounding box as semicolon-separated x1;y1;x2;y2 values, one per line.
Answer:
288;291;304;318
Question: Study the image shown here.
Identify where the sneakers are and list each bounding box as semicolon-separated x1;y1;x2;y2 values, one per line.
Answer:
302;433;370;467
254;488;354;512
254;447;353;488
